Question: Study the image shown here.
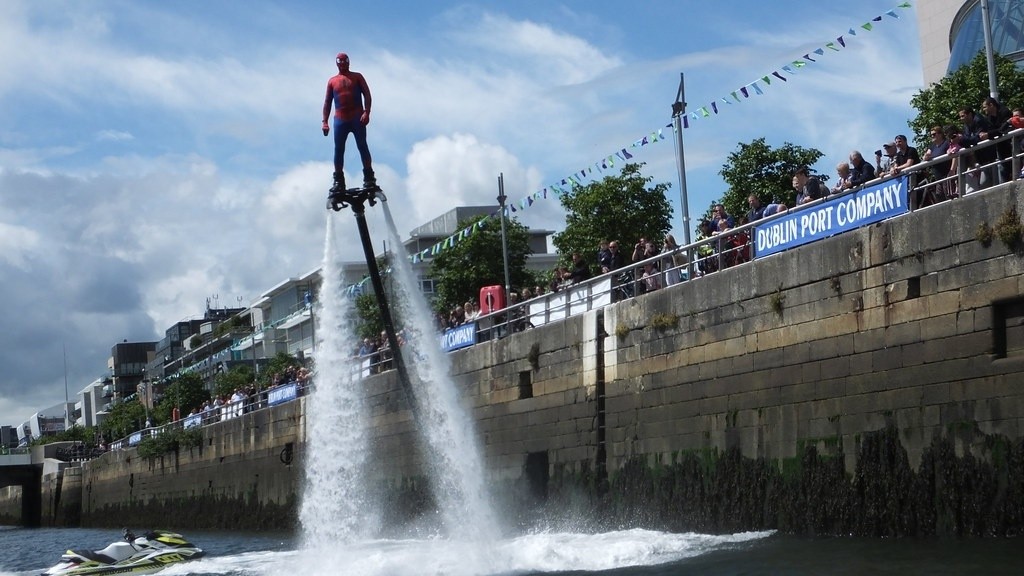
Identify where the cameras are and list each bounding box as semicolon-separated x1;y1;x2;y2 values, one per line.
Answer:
450;311;457;316
641;242;645;246
875;150;882;158
662;239;667;242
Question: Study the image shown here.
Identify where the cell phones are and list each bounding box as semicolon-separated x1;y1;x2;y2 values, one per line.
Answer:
951;135;956;139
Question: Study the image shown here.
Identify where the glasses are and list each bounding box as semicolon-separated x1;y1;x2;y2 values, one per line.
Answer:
609;246;616;249
884;146;891;149
930;132;938;137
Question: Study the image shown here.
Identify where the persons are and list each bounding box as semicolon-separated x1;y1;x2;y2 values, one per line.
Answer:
791;169;830;207
185;266;574;425
692;204;751;274
923;126;955;201
659;234;682;286
978;98;1014;183
830;162;855;194
599;239;627;304
890;134;925;209
843;150;877;189
631;238;660;295
943;125;981;194
762;203;788;219
122;527;135;543
873;142;898;178
951;107;999;189
746;194;764;222
560;252;592;284
999;107;1024;180
321;52;380;193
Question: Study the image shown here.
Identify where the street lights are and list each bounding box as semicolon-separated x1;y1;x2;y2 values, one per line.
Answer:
142;367;148;428
71;412;75;459
175;358;180;420
165;356;185;369
671;73;696;279
383;237;399;368
305;280;315;357
249;313;260;407
497;174;514;335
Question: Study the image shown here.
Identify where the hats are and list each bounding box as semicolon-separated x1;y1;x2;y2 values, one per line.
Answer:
883;142;895;146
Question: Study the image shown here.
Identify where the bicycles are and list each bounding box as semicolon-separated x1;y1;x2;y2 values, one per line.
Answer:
503;313;535;332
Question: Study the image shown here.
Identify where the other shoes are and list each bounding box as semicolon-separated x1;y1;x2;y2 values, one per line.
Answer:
329;171;346;192
947;192;959;200
363;172;377;187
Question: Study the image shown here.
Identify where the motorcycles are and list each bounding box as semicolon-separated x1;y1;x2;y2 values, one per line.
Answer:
38;530;204;576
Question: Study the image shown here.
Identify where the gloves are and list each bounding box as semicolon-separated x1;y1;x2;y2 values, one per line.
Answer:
360;111;370;126
322;122;330;137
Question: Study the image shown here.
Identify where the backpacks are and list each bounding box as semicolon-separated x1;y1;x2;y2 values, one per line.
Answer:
808;176;830;197
